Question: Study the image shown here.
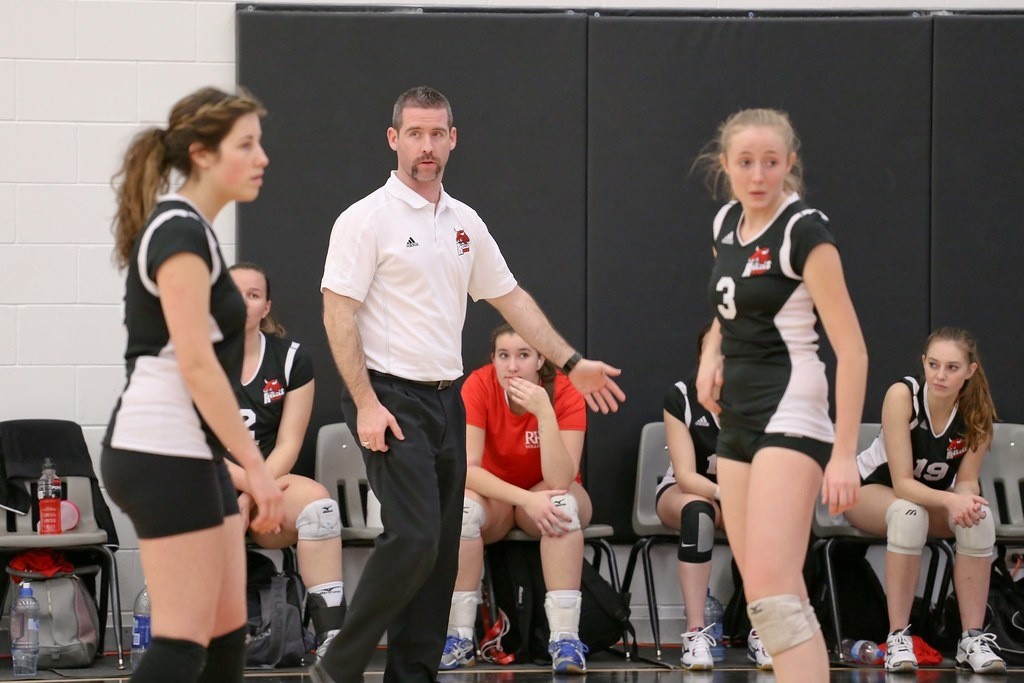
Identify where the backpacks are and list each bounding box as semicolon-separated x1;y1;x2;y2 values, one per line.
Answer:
247;572;316;670
490;541;635;662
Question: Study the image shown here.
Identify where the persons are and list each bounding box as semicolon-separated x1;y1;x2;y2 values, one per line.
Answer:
101;84;287;683
308;86;625;683
685;108;869;683
438;323;592;673
843;326;1007;674
654;374;775;670
223;264;347;668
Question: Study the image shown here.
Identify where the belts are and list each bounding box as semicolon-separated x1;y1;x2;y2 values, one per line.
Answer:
369;368;454;390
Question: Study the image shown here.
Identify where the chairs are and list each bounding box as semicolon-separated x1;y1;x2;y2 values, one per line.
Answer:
813;422;956;667
484;524;632;664
621;421;726;660
314;423;384;548
0;420;128;670
979;423;1024;667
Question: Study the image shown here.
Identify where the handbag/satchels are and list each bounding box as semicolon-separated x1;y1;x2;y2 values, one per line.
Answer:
4;561;100;669
940;561;1024;667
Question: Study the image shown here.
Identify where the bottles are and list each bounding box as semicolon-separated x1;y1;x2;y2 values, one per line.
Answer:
703;589;725;663
835;638;886;665
129;579;151;673
38;458;63;536
9;582;39;678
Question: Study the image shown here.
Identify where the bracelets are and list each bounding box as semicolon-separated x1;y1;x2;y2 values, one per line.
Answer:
561;352;582;376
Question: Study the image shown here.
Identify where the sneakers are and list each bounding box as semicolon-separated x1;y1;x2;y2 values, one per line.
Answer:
549;632;589;674
955;629;1006;673
884;624;919;671
680;623;718;670
746;629;771;669
440;636;475;670
